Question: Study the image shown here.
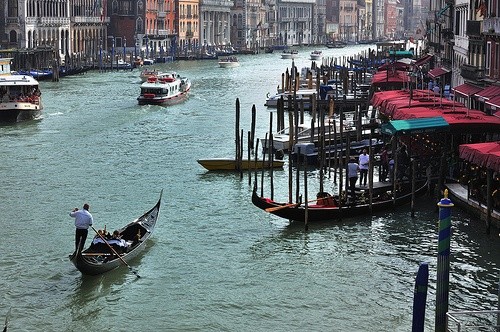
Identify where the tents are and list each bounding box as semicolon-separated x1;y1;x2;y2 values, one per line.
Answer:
370;70;419;88
370;91;500;134
457;142;500;172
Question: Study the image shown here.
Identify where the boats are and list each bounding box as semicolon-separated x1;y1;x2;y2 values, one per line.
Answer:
196;158;285;170
252;178;428;220
68;189;162;276
137;69;191;105
18;47;239;80
281;50;299;59
310;50;322;59
218;56;239;67
0;58;44;122
266;43;384;161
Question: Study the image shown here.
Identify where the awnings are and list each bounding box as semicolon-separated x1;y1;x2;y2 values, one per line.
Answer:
452;83;500;119
428;66;450;78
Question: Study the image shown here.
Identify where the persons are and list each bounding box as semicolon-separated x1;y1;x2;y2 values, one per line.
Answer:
69;203;93;255
357;149;370;189
398;145;409;161
18;85;41;102
346;159;362;198
418;77;441;94
380;147;390;183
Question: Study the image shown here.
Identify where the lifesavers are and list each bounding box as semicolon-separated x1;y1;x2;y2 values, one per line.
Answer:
25;98;33;103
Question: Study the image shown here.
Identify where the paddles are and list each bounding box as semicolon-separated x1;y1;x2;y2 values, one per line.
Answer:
265;195;339;212
90;225;141;278
